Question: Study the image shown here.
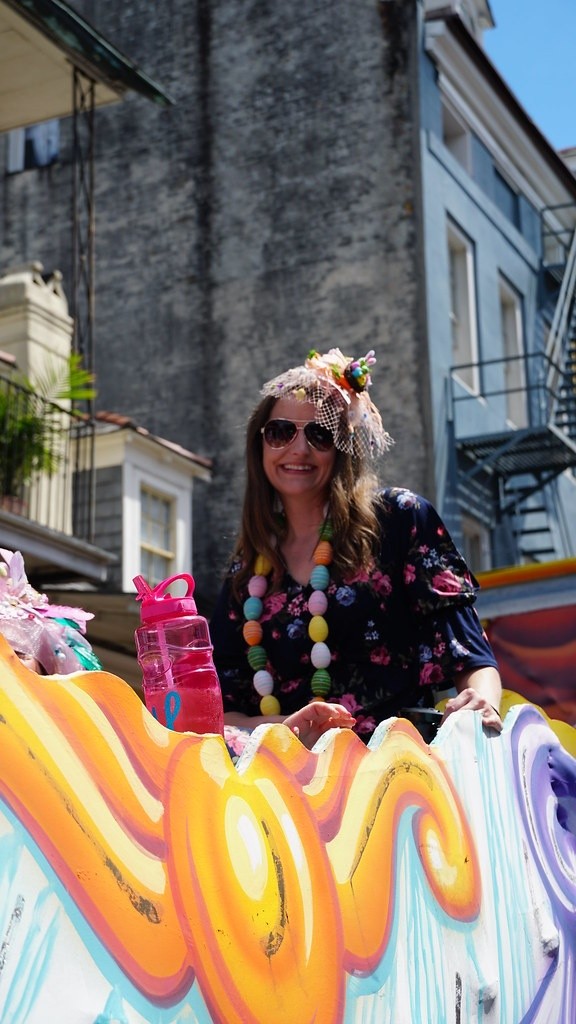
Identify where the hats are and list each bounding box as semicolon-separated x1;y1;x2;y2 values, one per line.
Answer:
0;547;103;673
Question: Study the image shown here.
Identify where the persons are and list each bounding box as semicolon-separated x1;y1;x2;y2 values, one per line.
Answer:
207;348;504;766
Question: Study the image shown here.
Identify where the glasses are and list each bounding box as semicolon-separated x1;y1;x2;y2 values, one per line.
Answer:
260;417;342;452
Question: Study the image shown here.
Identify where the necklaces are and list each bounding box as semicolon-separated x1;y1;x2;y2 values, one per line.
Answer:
243;519;334;715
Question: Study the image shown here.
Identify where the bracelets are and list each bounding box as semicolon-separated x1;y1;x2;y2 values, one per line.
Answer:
491;705;502;718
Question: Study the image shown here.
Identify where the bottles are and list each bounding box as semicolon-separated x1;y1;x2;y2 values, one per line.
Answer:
133;573;225;739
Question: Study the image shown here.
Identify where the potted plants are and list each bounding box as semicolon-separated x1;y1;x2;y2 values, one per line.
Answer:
0;347;99;516
